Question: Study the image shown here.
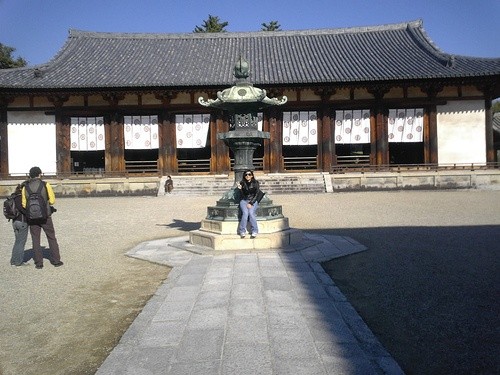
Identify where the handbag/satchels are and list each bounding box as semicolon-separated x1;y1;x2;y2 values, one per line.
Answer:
257;190;265;203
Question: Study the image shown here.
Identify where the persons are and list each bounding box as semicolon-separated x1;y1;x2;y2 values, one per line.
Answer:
21;167;64;269
10;181;28;266
165;175;174;192
235;169;259;238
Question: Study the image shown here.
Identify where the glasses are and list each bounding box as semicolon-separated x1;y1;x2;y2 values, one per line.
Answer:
245;174;251;176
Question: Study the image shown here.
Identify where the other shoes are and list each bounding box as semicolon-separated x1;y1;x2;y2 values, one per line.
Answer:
11;262;30;267
241;233;245;236
55;262;63;267
251;232;257;237
35;265;43;269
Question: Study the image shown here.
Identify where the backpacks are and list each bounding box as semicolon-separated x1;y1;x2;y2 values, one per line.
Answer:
3;194;22;222
25;181;48;225
